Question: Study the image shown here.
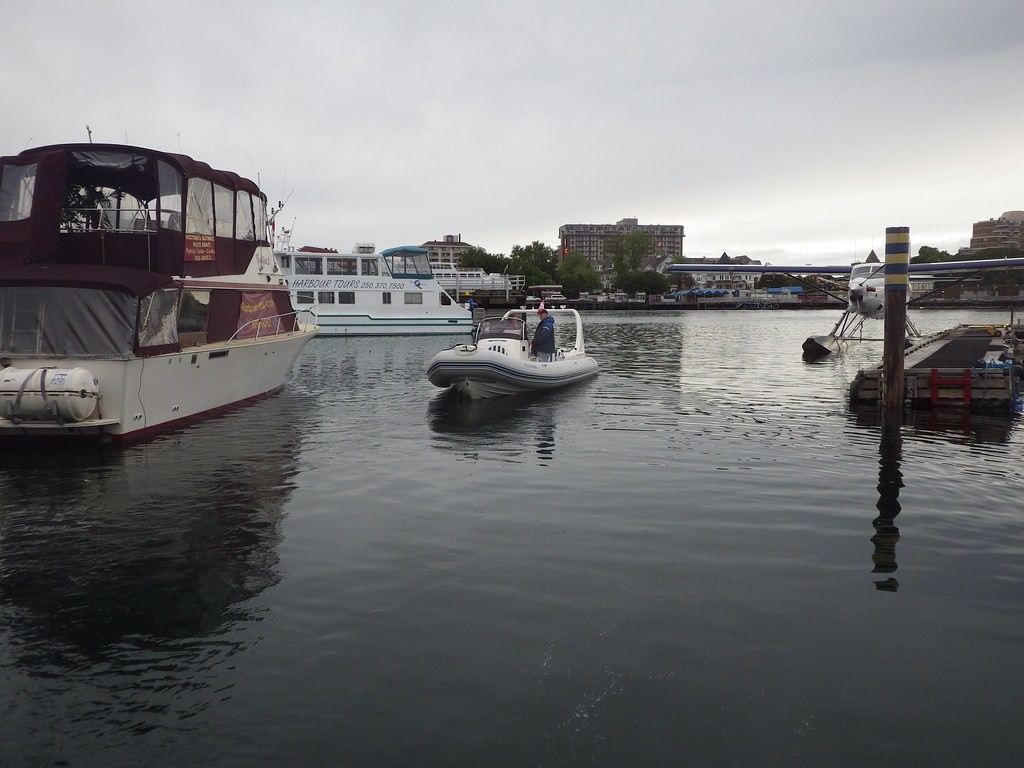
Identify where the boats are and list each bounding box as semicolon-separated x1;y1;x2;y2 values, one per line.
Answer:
426;303;598;401
0;125;321;444
273;216;490;338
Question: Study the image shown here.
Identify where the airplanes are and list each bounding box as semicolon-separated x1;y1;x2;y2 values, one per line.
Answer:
666;254;1024;358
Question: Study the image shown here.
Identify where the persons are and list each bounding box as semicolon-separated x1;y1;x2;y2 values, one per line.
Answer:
531;309;555;362
468;298;475;312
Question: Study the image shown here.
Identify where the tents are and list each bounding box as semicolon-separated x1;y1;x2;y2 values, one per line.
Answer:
672;288;730;302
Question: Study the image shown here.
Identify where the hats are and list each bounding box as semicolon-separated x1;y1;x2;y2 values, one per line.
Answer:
537;309;548;314
